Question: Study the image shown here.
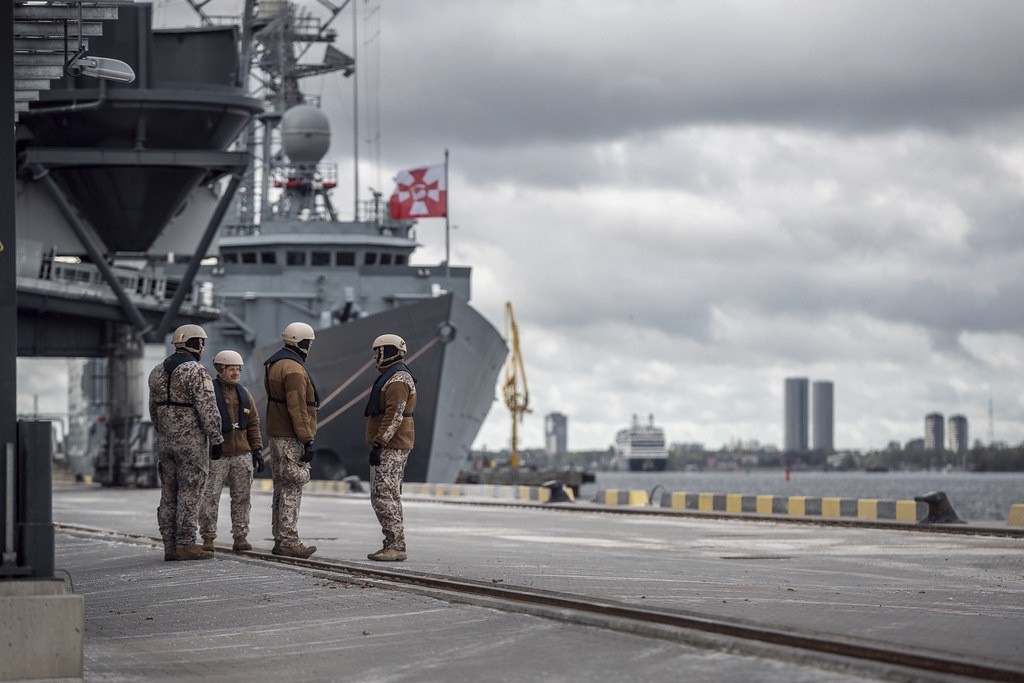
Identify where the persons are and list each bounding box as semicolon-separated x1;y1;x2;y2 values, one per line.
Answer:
263;321;321;557
364;334;420;561
148;324;225;561
197;349;264;550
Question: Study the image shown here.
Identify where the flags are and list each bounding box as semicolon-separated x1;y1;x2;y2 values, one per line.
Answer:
384;162;447;221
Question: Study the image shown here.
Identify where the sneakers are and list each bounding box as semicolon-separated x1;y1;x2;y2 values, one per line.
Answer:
202;538;215;552
174;544;214;561
165;546;177;560
367;548;407;561
271;541;317;559
232;536;252;551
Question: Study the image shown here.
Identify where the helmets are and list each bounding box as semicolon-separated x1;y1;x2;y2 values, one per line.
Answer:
172;324;208;344
372;334;407;354
213;350;243;365
282;322;315;347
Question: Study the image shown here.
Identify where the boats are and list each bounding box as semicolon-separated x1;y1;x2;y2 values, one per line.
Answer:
66;0;512;486
458;447;598;504
612;410;670;472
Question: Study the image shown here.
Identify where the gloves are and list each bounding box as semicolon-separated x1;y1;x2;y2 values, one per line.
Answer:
252;449;265;473
300;441;314;463
369;441;383;466
211;444;223;460
